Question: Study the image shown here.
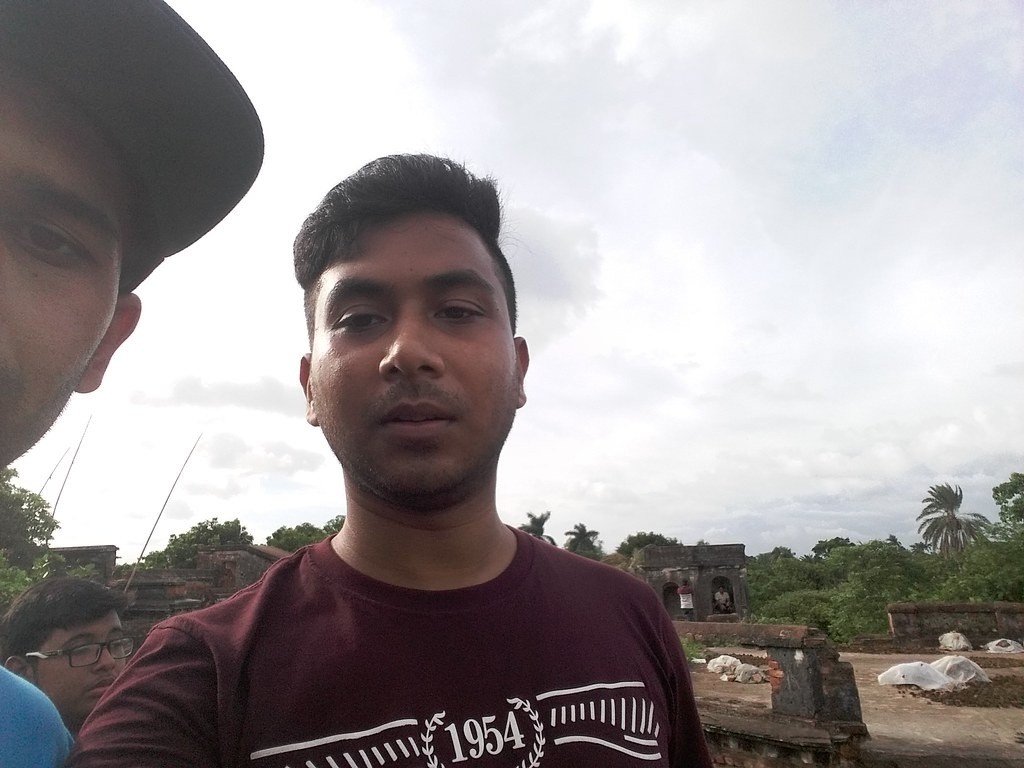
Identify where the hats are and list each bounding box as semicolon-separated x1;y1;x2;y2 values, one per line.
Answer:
0;0;267;296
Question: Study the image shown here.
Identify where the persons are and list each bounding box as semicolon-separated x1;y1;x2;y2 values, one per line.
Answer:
0;575;135;738
714;586;730;611
0;0;266;768
63;154;713;768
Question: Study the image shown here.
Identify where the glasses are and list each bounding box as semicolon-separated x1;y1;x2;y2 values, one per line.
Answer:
25;635;134;668
